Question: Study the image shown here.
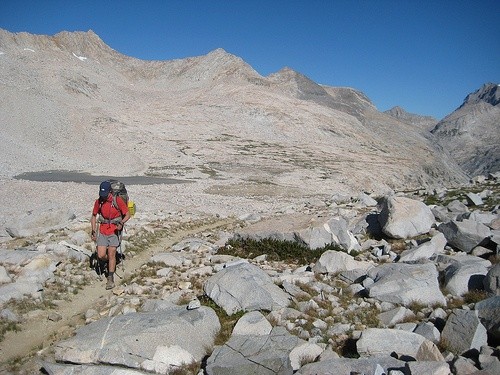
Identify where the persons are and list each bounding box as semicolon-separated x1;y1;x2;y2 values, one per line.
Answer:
91;181;130;290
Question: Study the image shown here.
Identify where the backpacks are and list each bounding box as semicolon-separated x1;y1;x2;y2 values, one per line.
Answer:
105;180;129;215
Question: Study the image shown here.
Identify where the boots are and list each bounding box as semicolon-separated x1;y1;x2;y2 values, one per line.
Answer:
106;276;115;289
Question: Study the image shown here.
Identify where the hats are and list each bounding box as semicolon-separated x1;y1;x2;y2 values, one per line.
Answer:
99;181;111;197
